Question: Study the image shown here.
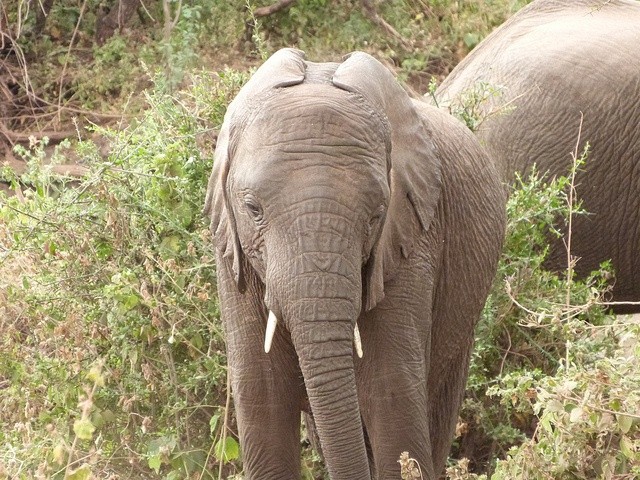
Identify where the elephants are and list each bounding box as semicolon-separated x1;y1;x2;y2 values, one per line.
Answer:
200;44;509;480
424;1;638;316
